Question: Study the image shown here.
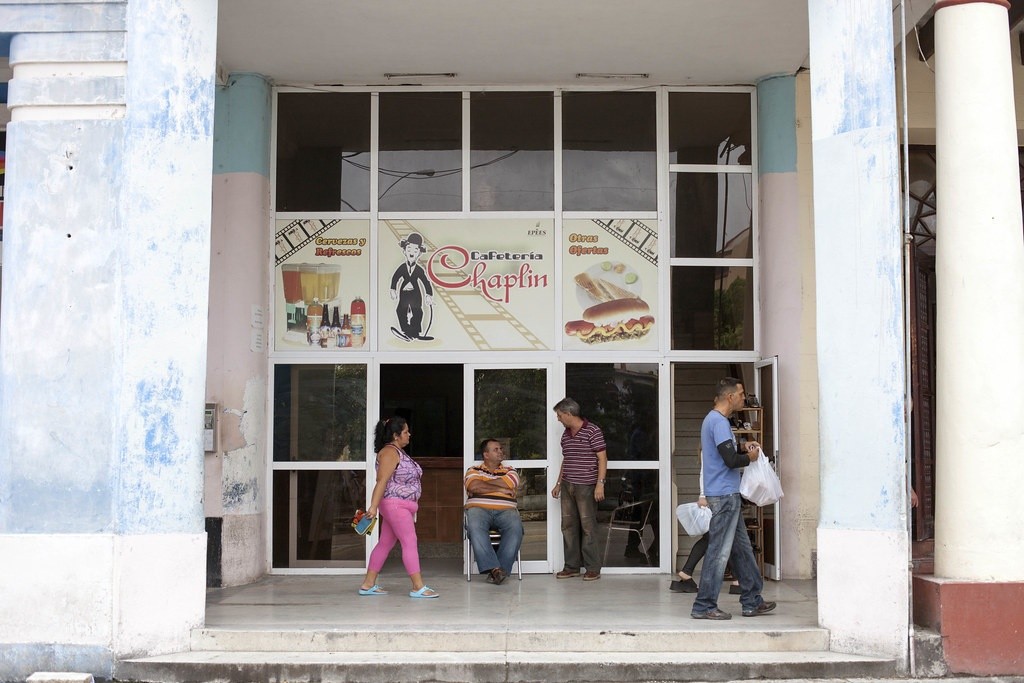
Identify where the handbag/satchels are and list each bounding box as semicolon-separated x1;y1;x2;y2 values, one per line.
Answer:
676;502;712;537
739;447;785;506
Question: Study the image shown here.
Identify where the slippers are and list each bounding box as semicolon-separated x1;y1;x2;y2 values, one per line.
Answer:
410;585;440;598
358;585;389;594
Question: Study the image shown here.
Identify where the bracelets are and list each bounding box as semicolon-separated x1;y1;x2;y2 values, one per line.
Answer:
557;482;561;484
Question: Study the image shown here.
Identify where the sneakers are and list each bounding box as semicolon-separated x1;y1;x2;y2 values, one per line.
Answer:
742;602;776;616
692;608;732;620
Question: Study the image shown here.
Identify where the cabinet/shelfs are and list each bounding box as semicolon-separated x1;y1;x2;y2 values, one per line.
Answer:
723;405;765;581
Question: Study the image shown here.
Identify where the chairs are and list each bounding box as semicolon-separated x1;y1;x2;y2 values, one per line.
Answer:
464;508;522;582
602;493;658;567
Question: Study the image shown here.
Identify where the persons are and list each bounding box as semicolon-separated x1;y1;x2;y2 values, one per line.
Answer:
463;438;522;585
690;377;777;621
623;413;659;562
359;418;440;598
552;397;607;581
669;428;744;594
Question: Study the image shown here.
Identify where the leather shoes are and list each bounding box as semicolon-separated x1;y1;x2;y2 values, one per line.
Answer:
491;568;506;585
488;572;496;584
557;570;579;578
583;571;600;580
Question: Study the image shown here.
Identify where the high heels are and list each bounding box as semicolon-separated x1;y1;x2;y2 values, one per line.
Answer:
729;585;742;594
670;578;699;592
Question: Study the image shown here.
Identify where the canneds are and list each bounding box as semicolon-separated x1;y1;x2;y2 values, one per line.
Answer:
350;325;363;348
308;326;320;346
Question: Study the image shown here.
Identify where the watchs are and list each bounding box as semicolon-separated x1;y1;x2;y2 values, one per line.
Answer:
597;478;606;483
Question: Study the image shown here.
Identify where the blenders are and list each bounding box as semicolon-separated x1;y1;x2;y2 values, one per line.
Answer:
281;261;342;342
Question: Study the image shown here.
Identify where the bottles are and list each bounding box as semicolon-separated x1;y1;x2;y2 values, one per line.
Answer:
306;295;366;347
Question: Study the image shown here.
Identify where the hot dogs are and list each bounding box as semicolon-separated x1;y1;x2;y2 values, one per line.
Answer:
565;297;655;344
